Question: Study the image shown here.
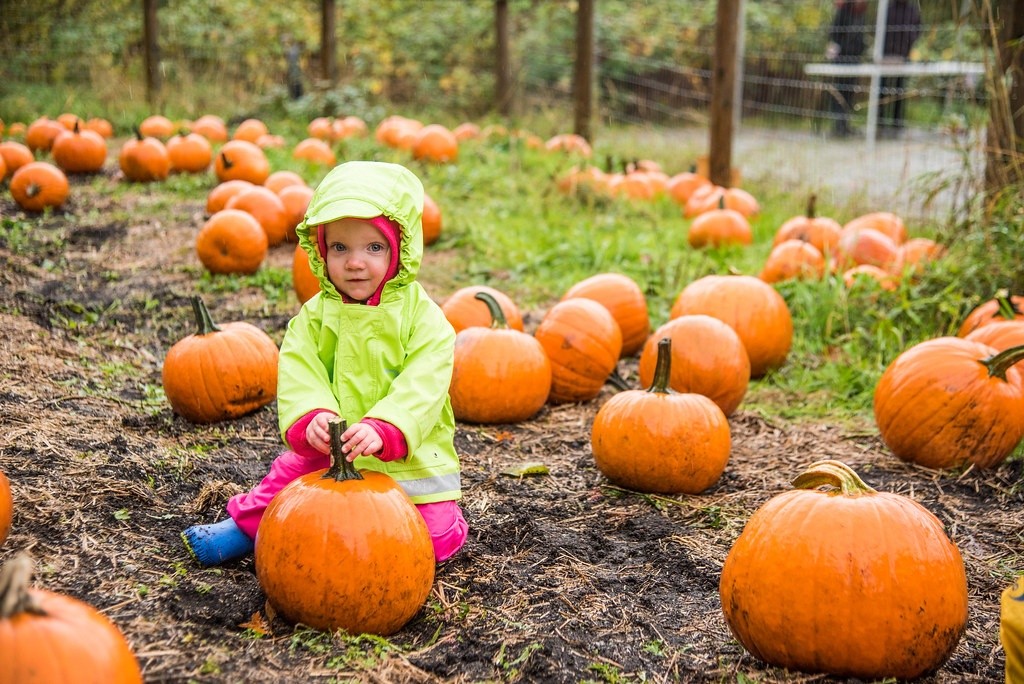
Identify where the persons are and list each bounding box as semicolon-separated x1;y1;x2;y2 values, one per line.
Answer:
824;0;922;138
181;161;470;563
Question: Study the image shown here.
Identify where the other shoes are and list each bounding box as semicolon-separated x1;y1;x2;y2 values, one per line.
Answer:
180;516;254;568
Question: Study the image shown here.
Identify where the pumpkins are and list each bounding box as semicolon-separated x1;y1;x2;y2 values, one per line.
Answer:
163;296;280;425
0;112;369;307
254;418;434;638
713;460;968;678
376;114;1024;492
0;468;144;684
